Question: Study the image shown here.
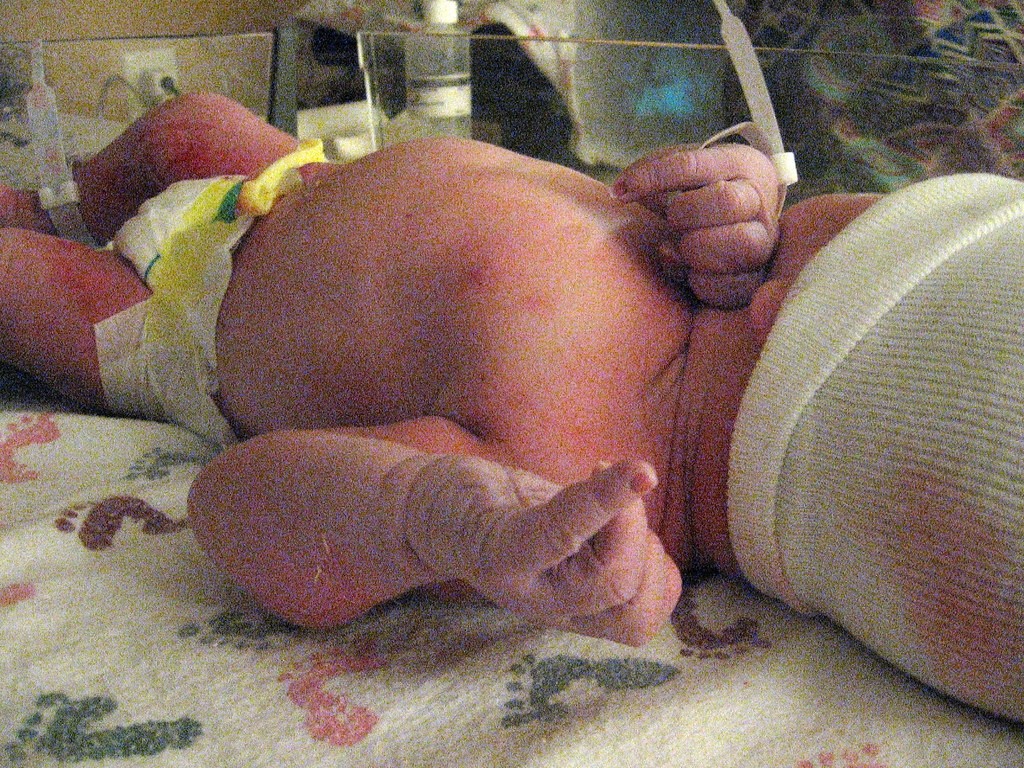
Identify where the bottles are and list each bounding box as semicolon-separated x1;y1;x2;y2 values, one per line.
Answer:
405;0;474;138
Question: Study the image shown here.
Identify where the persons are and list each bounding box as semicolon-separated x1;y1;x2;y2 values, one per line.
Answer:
0;91;1024;727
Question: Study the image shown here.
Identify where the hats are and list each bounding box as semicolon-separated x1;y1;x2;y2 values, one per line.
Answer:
724;171;1023;726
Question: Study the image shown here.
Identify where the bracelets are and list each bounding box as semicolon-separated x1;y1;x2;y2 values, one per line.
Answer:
700;0;800;221
